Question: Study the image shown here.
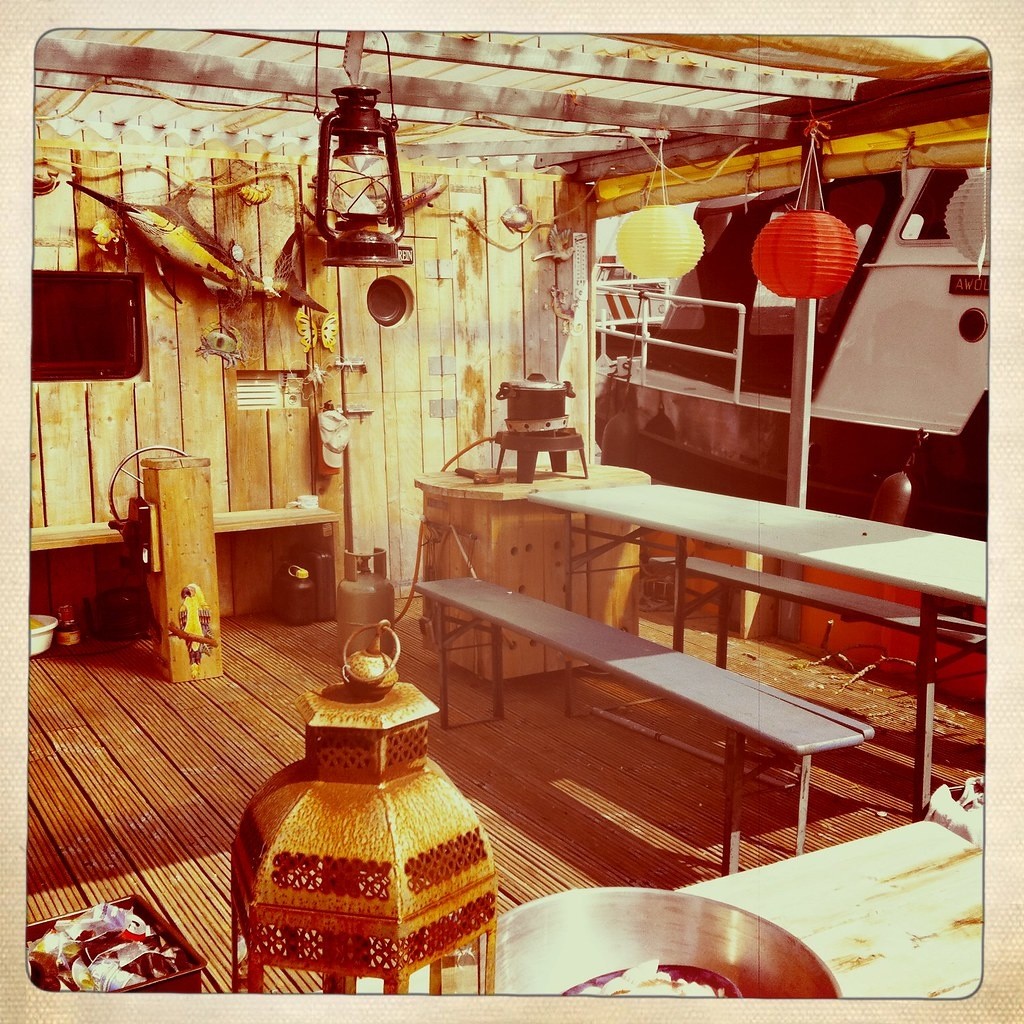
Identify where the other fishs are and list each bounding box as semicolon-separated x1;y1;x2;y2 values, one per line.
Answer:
501;204;532;233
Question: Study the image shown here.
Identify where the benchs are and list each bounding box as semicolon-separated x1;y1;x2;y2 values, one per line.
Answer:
414;576;876;877
651;556;987;671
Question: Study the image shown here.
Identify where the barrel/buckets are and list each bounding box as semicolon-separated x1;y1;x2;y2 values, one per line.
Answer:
271;560;314;624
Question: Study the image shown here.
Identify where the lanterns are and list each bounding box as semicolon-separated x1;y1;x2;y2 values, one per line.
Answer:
615;204;706;279
944;168;991;268
750;210;860;300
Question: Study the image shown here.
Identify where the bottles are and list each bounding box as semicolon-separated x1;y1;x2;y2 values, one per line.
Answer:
55;604;81;650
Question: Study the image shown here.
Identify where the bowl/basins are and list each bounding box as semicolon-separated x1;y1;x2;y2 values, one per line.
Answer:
436;887;844;997
297;494;318;509
28;614;58;657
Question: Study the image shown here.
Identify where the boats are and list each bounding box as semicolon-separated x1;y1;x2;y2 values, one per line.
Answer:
593;166;988;545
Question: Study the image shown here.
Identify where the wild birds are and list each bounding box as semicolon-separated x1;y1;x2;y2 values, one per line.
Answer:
531;223;576;261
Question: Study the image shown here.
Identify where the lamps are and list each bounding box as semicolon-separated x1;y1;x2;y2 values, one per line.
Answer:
314;30;404;266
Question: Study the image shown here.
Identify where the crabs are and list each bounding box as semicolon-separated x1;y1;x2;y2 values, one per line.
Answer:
193;316;245;370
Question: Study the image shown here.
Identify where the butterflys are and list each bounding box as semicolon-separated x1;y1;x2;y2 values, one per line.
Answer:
294;307;339;354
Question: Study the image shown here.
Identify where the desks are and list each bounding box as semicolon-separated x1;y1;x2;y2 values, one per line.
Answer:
30;508;341;619
528;484;988;827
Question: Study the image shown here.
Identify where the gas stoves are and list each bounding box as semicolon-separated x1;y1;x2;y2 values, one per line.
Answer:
492;426;590;484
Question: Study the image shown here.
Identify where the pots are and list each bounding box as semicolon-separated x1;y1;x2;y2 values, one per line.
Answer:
495;373;576;420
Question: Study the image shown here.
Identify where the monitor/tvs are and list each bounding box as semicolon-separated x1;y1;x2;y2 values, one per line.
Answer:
33;275;140;382
